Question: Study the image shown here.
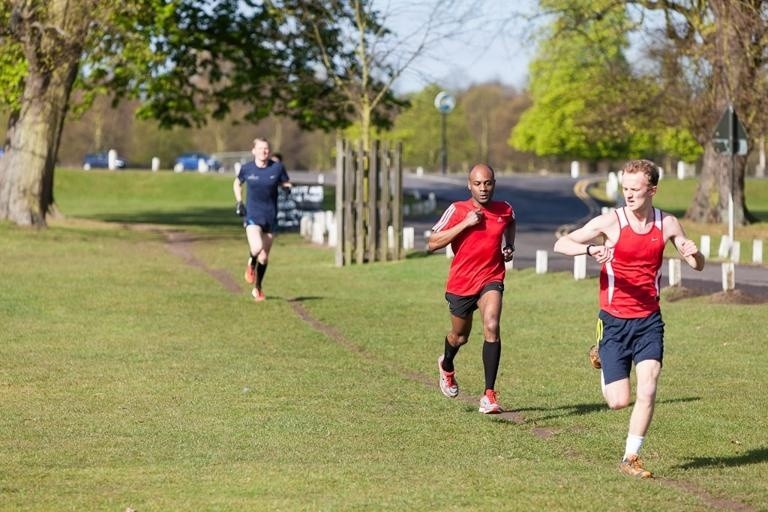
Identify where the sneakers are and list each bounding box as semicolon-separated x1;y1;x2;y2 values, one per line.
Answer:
478;389;504;414
589;346;602;368
618;452;651;480
252;287;264;302
244;257;256;284
437;355;459;398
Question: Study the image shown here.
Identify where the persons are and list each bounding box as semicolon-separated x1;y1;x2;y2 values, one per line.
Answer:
553;159;705;480
428;163;516;415
233;137;293;301
272;152;282;165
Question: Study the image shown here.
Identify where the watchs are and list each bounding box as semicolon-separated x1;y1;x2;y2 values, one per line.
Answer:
587;242;596;257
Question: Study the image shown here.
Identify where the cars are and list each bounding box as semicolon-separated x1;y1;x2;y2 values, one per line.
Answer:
82;151;130;170
174;151;222;172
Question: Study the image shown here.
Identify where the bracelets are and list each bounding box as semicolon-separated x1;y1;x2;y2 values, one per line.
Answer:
504;244;516;252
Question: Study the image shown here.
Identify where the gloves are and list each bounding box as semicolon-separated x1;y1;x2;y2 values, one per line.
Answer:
235;202;246;217
